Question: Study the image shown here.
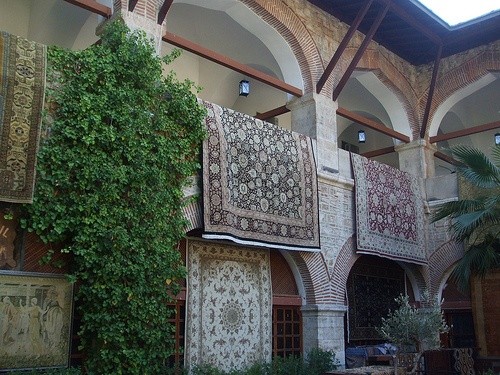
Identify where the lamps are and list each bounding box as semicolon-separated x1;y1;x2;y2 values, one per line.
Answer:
239;80;250;97
358;129;366;143
495;133;500;145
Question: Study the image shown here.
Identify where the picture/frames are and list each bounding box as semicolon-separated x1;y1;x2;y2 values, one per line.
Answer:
0;269;75;374
